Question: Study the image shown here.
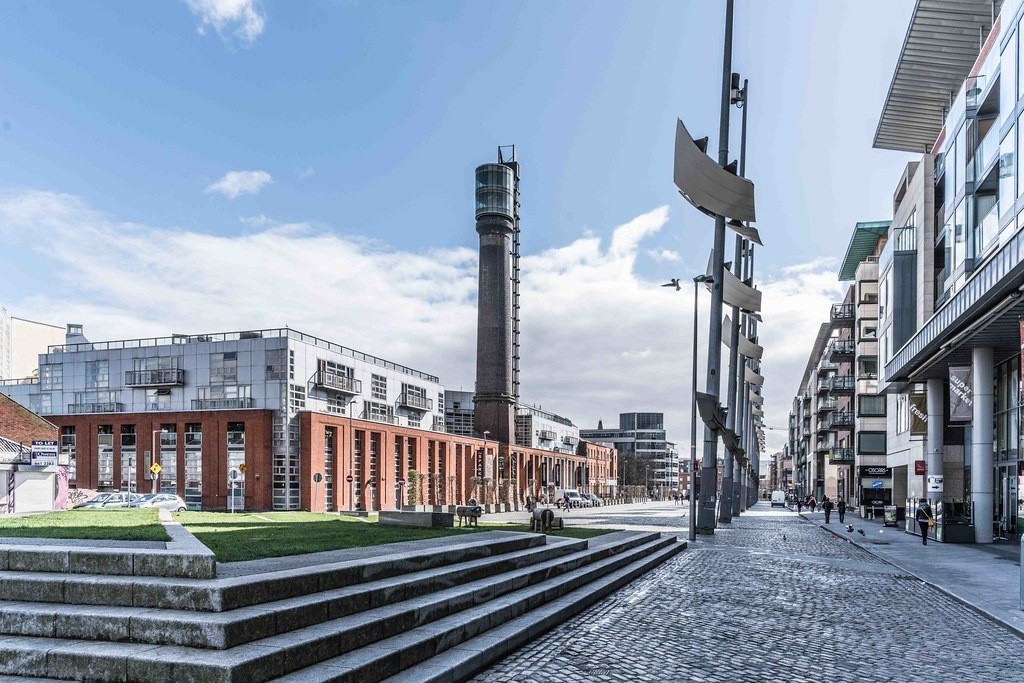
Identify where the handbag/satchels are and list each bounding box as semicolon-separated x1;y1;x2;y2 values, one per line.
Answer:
928;517;934;527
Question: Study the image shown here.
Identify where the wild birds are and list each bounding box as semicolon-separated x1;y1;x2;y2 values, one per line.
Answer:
783;535;786;541
661;278;681;291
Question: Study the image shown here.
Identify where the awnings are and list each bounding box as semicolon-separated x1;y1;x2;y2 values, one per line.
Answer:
862;477;892;488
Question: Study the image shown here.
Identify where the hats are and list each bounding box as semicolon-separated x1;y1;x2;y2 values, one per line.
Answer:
920;498;926;502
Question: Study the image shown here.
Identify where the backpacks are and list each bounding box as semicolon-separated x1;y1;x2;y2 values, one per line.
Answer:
838;502;844;513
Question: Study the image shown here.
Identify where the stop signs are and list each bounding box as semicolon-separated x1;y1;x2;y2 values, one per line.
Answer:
346;475;353;482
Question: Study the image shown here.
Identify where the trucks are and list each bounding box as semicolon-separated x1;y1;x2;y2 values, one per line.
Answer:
554;490;582;508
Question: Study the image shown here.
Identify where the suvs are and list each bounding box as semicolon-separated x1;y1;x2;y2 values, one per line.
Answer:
579;493;601;506
72;494;143;509
123;493;187;512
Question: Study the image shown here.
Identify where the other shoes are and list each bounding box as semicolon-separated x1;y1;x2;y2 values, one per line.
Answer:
924;541;927;545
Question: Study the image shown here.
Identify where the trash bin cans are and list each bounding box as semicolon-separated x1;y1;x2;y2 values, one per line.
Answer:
1018;533;1024;614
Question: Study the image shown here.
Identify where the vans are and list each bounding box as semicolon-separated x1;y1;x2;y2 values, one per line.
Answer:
771;490;785;507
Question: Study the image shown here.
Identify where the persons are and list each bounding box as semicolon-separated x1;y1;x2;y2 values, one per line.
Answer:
468;494;476;522
915;499;932;545
625;491;628;499
527;495;531;510
790;496;793;502
804;495;816;512
837;498;845;523
768;493;772;501
680;494;684;504
532;497;536;507
785;494;789;500
563;495;570;512
621;492;623;497
822;497;833;523
822;495;826;503
541;498;544;504
675;494;679;505
796;497;803;512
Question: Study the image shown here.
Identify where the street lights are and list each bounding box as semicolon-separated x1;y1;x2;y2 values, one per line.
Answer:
349;400;358;510
484;431;490;502
151;429;168;494
623;460;627;496
688;274;714;539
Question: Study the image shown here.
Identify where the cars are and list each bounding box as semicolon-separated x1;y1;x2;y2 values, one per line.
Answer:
580;496;591;507
788;494;797;501
